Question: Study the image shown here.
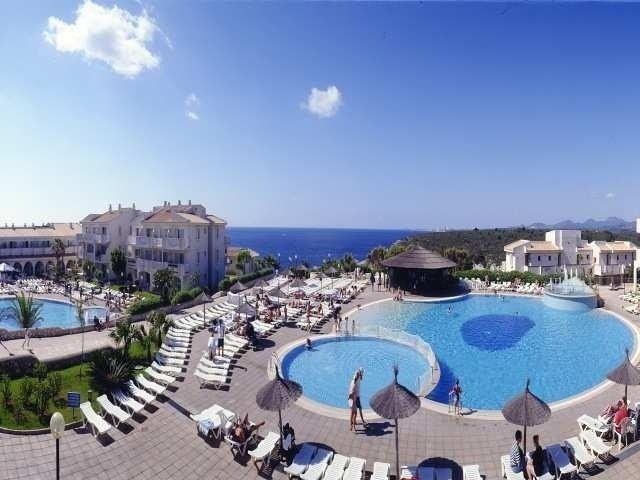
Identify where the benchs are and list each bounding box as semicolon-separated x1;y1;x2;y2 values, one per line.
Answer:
370;462;391;480
400;464;417;479
462;464;482;480
321;453;366;480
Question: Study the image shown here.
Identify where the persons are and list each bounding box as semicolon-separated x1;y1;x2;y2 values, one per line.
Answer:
447;378;463;416
370;273;375;289
347;370;359;433
21;325;33;350
350;367;367;424
509;429;529;473
64;278;128;332
397;285;404;300
206;284;360;358
285;423;295;448
377;273;382;292
281;424;295;467
610;396;628;434
229;410;266;444
600;400;622;419
526;433;545;480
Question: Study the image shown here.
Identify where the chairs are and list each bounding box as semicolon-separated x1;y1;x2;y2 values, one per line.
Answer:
174;301;275;390
187;403;281;480
80;325;191;440
283;444;334;480
469;277;543;295
500;403;639;479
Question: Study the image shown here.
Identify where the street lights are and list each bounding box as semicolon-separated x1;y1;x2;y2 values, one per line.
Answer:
50;412;64;480
278;253;280;265
289;256;297;266
328;253;331;262
